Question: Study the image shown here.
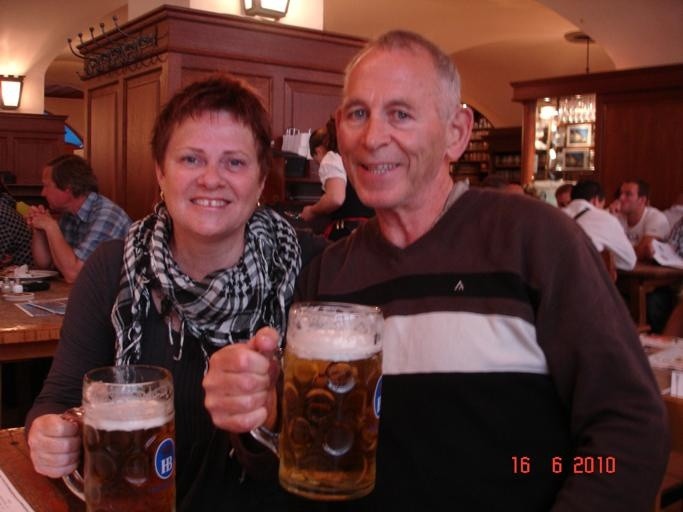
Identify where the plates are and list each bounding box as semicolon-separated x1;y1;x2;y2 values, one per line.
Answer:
2;292;35;302
5;269;59;284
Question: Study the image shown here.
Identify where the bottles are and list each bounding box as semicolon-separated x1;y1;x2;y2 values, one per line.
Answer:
12;277;24;294
1;276;12;294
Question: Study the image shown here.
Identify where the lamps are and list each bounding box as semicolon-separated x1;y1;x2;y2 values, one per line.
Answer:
1;74;25;110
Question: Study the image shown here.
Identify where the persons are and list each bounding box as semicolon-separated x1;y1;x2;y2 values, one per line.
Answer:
480;174;680;337
0;186;35;270
25;74;341;511
295;126;376;239
198;28;672;512
19;153;132;285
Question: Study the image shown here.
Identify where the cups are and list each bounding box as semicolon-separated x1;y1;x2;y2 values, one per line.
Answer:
251;301;385;501
62;365;176;512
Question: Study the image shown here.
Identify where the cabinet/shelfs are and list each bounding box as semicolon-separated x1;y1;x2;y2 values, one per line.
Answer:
450;128;523;186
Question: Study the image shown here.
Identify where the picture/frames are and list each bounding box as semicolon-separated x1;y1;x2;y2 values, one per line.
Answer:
563;123;592;172
